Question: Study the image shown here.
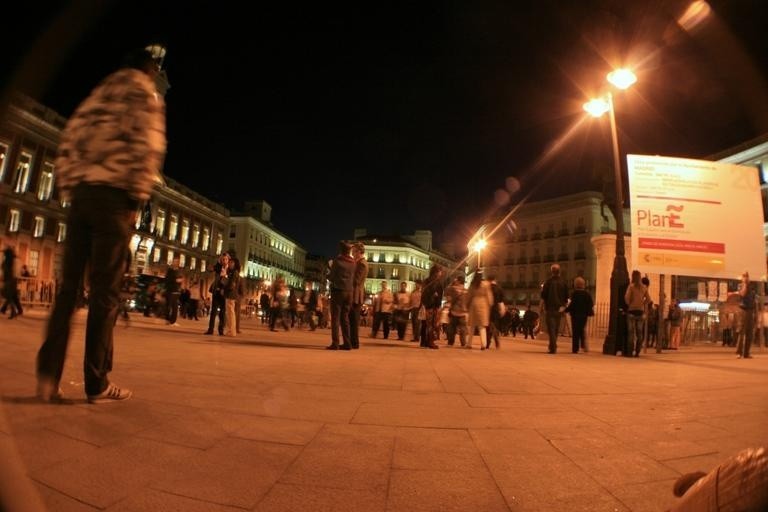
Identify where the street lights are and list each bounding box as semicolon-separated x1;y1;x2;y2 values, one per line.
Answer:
585;65;637;352
472;239;488;270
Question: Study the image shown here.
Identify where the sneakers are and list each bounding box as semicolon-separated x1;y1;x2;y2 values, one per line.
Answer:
542;345;681;358
36;379;64;402
85;383;132;404
0;307;23;319
370;330;503;352
142;311;242;339
261;320;360;351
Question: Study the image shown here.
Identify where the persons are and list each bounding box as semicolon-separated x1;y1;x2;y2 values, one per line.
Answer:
36;46;174;402
